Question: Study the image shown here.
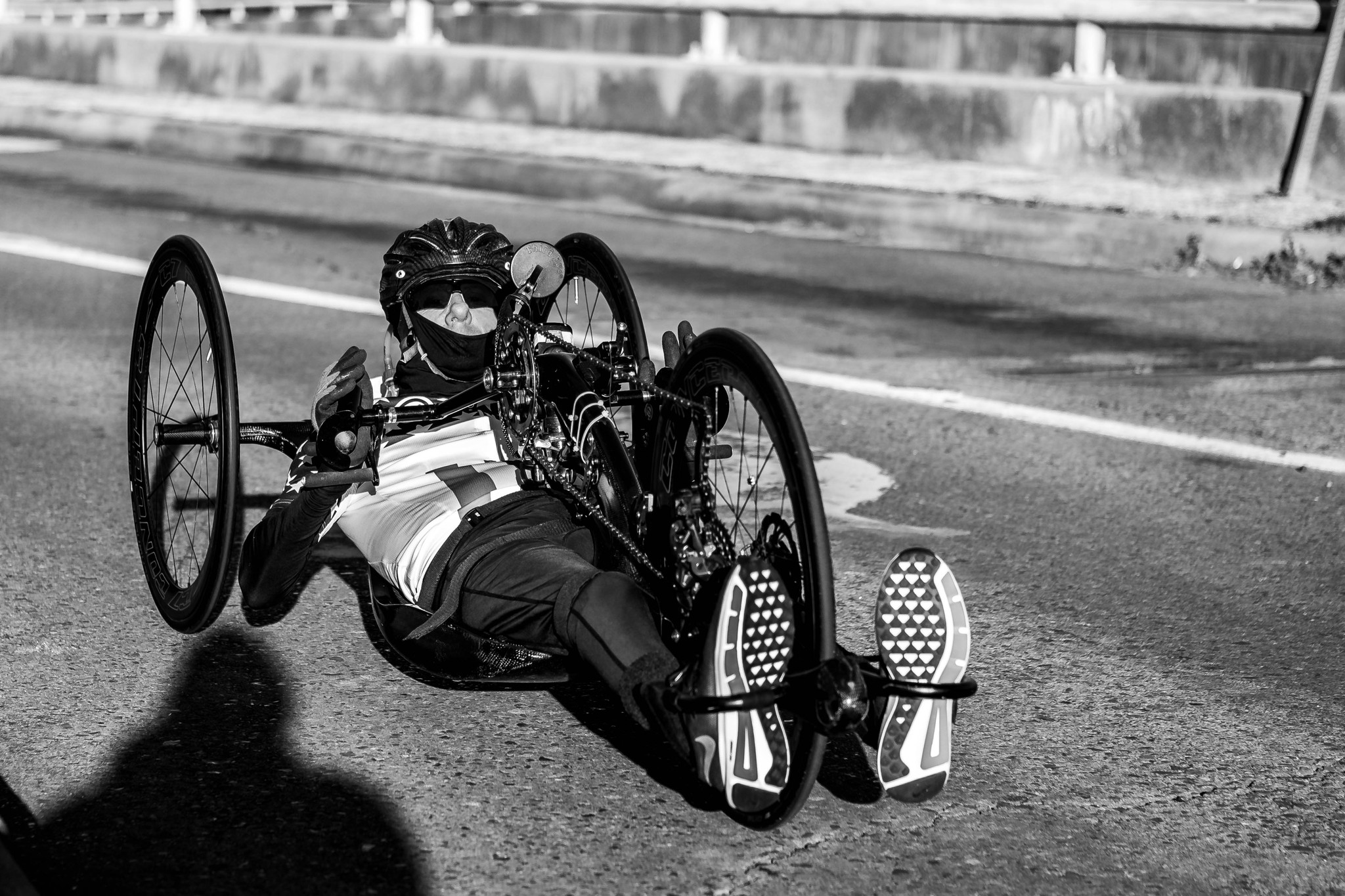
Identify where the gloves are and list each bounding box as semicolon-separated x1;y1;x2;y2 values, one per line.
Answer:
312;345;375;470
639;319;714;444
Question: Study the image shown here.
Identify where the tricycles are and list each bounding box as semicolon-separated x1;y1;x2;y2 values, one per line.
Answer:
127;230;979;833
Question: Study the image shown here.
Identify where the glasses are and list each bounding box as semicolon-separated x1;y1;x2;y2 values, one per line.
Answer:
406;279;498;310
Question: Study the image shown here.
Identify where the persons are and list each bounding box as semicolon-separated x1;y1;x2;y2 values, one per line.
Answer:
239;218;973;807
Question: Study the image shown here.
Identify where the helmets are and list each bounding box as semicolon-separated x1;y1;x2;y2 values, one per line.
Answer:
379;218;514;321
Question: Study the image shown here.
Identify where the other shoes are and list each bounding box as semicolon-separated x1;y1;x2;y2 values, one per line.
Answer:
874;546;972;802
669;557;806;813
